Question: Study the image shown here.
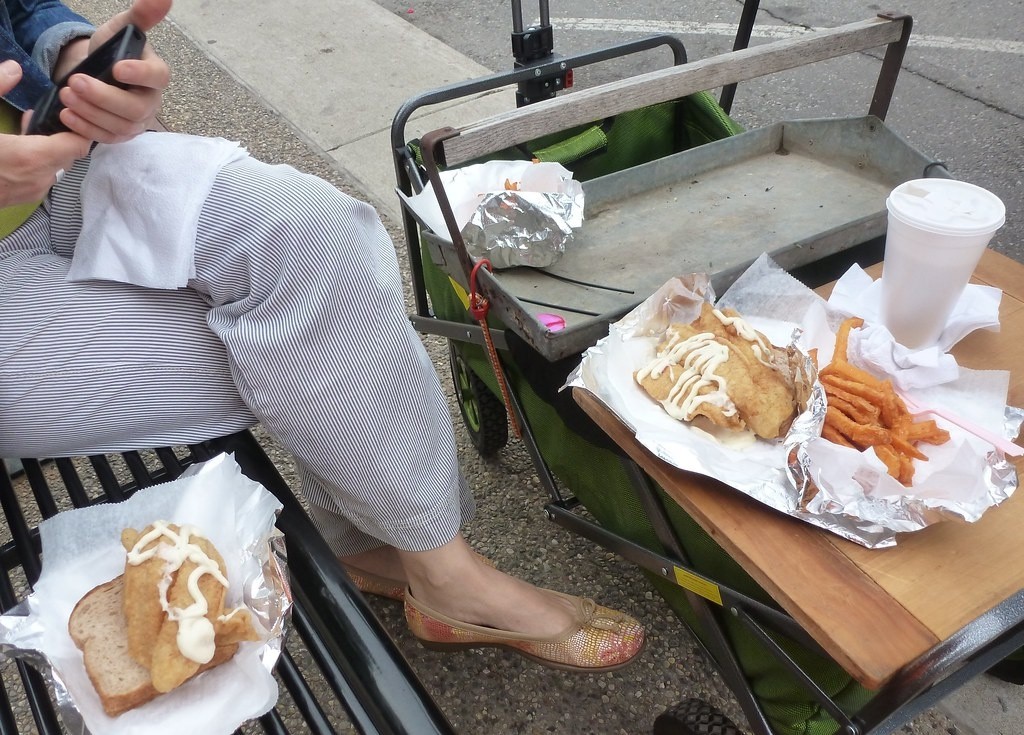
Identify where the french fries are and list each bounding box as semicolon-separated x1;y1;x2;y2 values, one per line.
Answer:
809;313;951;494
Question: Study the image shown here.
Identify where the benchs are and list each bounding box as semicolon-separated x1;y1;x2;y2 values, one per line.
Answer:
0;427;457;735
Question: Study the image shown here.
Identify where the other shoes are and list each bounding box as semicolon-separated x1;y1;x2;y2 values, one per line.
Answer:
403;581;647;672
337;548;498;601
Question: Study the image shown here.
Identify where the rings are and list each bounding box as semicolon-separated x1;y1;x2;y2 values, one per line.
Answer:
56;168;65;182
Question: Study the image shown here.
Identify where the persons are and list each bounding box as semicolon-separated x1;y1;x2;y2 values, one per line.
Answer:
0;0;644;677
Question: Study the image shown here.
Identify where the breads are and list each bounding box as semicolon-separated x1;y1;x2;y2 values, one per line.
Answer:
66;572;240;717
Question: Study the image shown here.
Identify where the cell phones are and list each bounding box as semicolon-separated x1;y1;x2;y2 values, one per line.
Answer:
26;24;147;139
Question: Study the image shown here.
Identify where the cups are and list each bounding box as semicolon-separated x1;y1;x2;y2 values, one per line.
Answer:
880;177;1006;351
